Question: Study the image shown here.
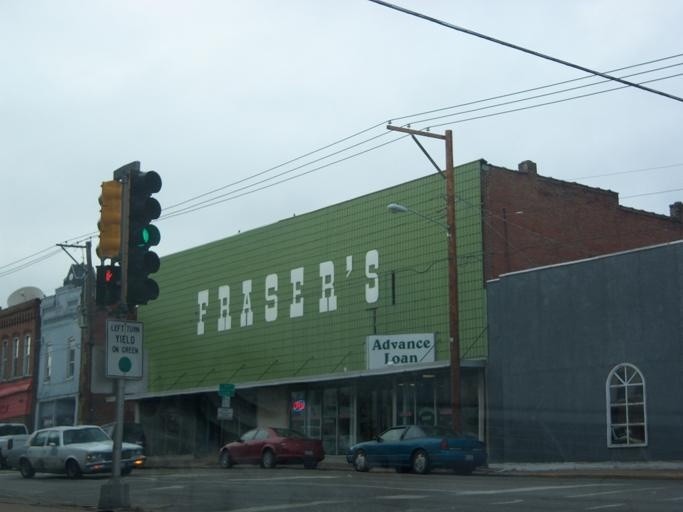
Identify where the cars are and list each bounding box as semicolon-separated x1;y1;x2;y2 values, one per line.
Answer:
6;426;146;480
347;425;486;475
219;426;325;469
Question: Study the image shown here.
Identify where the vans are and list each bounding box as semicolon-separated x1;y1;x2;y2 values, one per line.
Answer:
1;422;31;467
97;421;146;456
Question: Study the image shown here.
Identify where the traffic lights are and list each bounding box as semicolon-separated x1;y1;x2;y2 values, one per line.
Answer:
96;180;122;259
128;170;162;308
96;267;120;306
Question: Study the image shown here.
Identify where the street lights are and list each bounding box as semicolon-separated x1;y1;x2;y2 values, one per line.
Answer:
389;203;461;262
